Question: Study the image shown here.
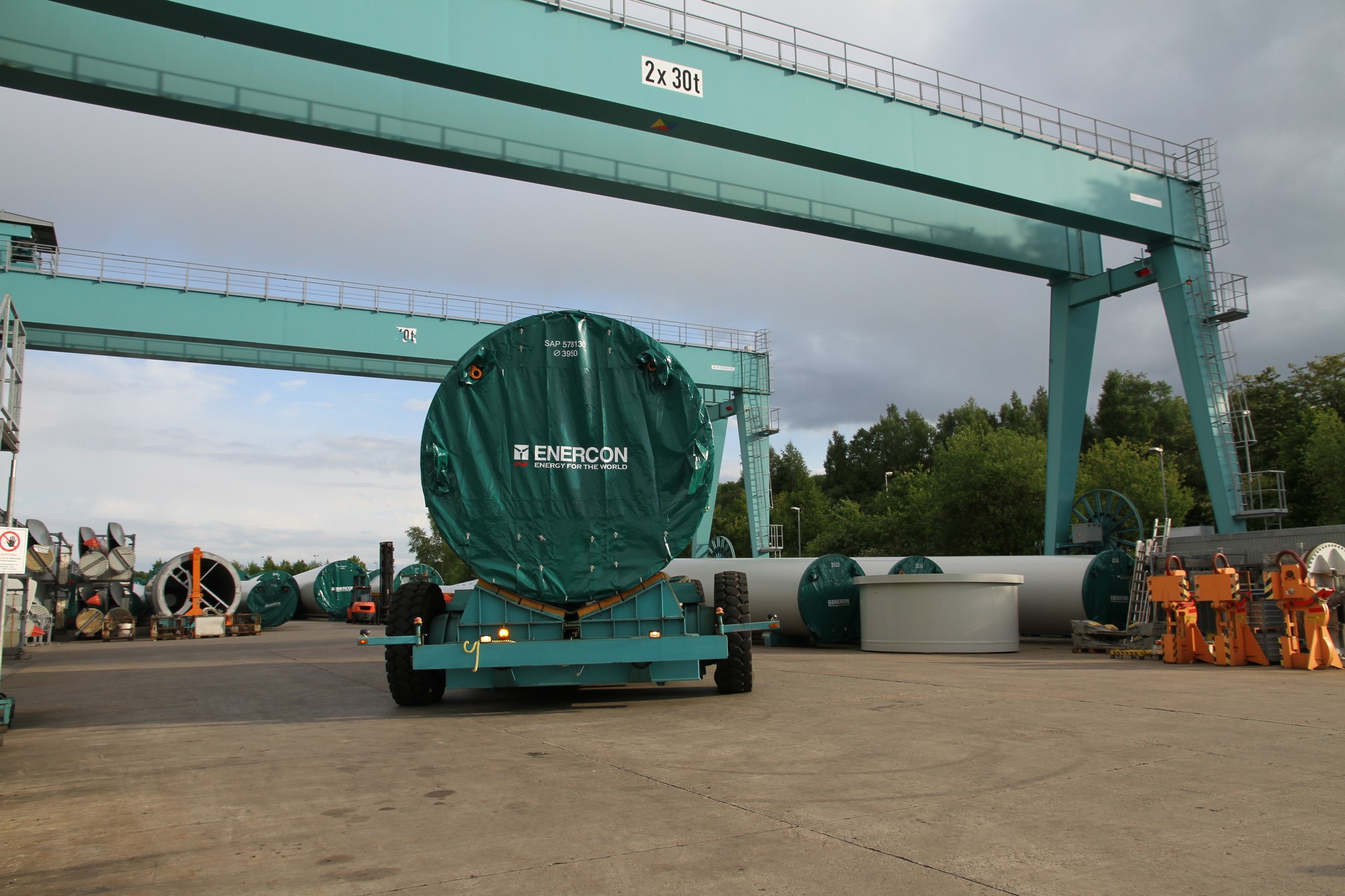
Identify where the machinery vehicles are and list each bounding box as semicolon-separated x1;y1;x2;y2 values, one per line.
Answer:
346;575;376;624
355;309;780;709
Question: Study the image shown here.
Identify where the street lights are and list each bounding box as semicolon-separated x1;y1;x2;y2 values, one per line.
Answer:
374;562;377;569
885;472;893;498
790;507;801;558
313;555;319;568
261;556;264;567
1148;447;1168;520
